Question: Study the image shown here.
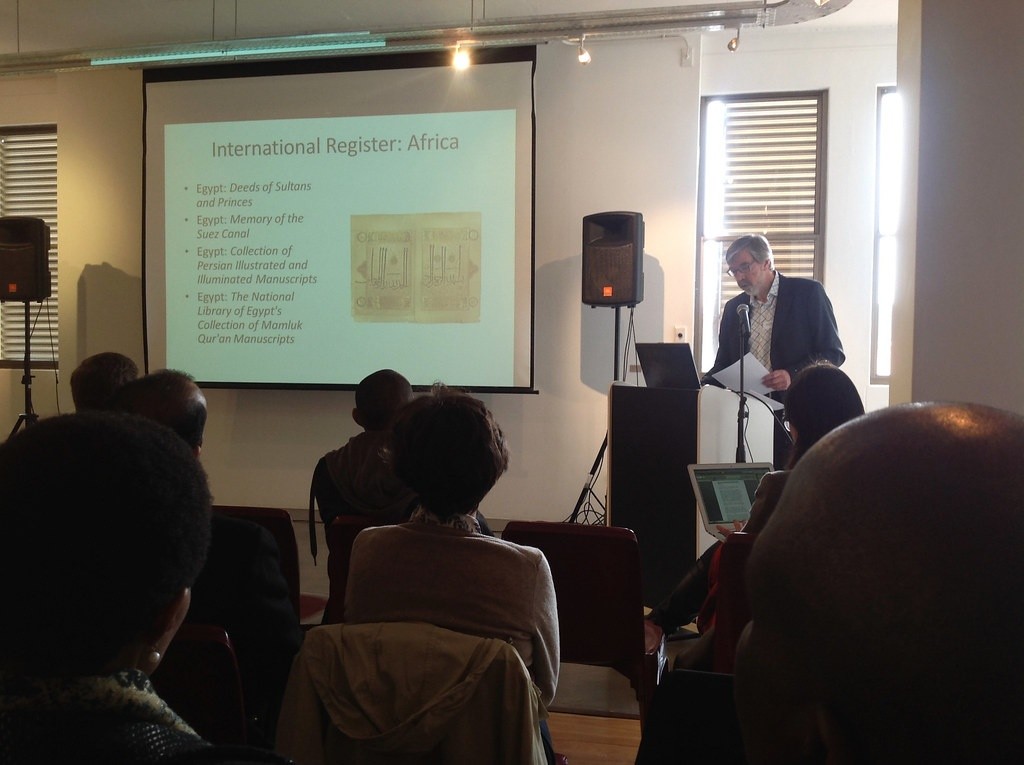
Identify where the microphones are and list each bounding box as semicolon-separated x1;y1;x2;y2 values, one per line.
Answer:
737;304;751;338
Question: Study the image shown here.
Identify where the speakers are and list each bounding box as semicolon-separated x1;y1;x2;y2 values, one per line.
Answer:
0;215;51;303
580;210;644;309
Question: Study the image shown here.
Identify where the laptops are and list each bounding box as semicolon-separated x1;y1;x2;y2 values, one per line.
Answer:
635;342;702;391
687;462;774;541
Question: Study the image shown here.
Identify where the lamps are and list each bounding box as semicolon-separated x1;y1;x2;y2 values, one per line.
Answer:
727;26;740;53
578;37;591;66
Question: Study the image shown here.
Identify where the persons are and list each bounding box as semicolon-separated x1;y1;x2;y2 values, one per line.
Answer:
71;351;137;414
0;410;271;764
643;362;865;659
112;371;307;746
734;402;1023;765
307;370;421;627
700;235;845;463
341;393;569;764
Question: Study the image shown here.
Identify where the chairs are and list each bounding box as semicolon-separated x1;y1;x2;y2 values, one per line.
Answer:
149;505;746;765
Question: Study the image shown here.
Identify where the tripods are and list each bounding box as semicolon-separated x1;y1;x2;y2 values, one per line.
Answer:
9;302;39;439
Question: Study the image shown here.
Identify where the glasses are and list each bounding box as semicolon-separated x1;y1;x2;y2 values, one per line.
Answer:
726;260;756;277
